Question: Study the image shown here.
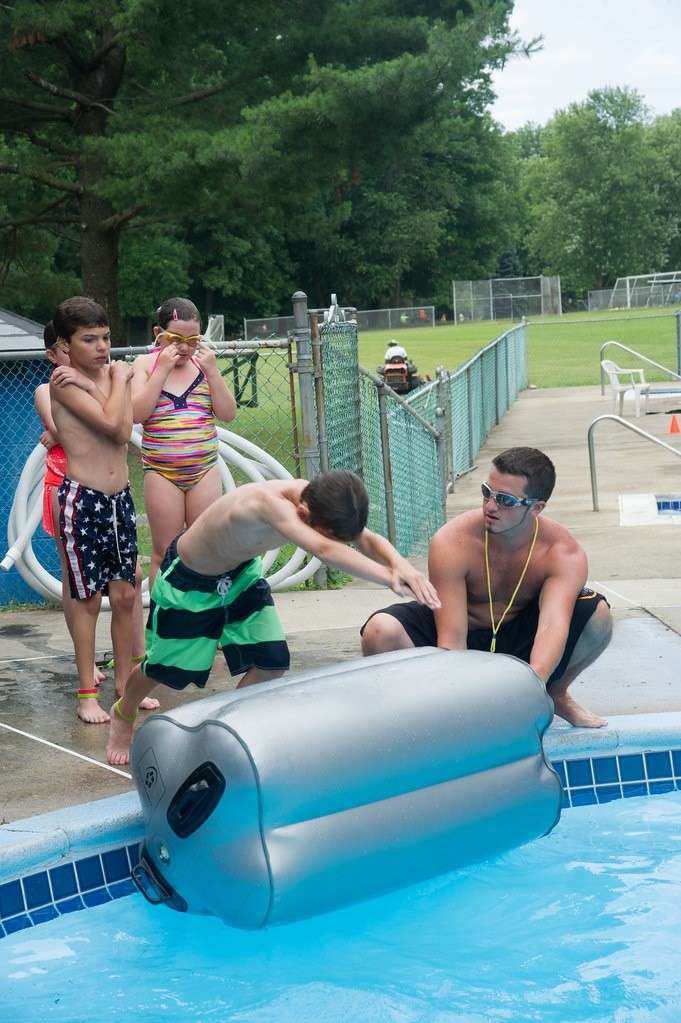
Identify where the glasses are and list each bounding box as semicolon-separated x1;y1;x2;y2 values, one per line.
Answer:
480;481;541;509
158;326;202;347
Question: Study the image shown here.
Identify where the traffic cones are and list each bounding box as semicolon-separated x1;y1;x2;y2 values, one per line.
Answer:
670;415;680;434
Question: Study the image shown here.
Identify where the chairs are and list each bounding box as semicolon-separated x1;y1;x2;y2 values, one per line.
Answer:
601;360;650;418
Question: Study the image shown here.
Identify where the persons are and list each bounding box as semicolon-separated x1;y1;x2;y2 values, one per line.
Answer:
384;339;409;365
399;313;409;324
361;445;615;729
106;470;443;766
34;316;148;686
49;295;160;724
131;295;237;650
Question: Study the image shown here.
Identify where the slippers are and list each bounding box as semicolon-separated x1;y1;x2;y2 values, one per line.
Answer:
95;650;114;666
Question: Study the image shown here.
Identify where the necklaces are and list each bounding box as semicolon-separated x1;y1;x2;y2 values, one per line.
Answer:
486;516;539;653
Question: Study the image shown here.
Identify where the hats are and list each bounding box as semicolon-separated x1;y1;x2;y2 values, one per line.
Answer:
388;340;397;345
